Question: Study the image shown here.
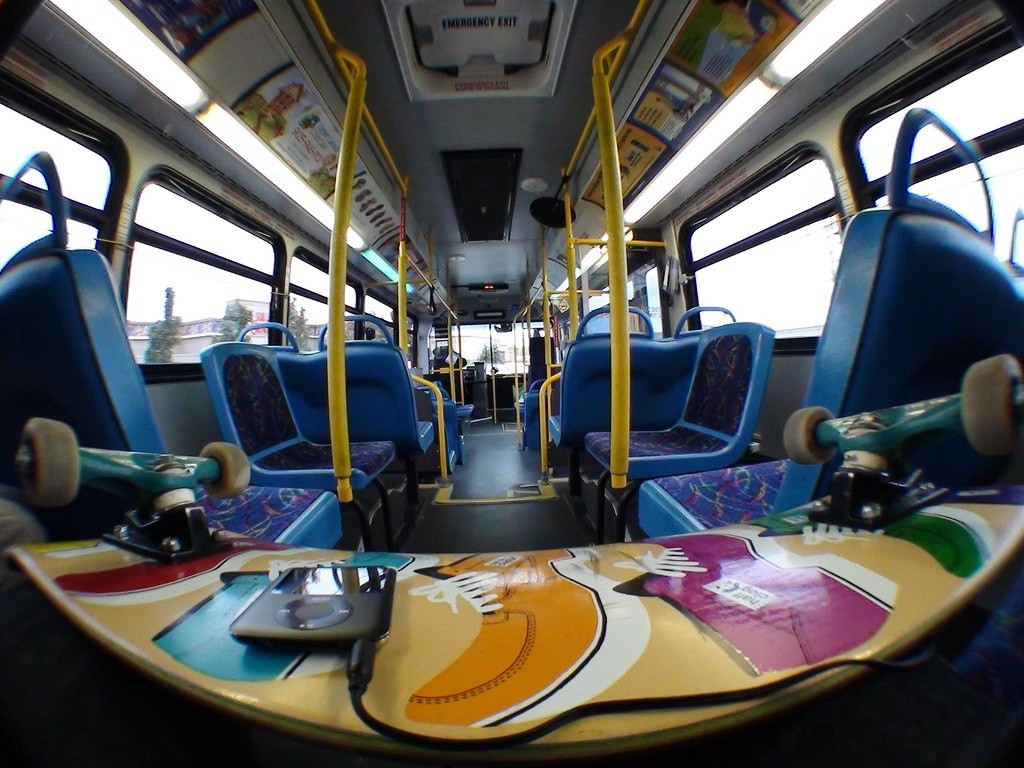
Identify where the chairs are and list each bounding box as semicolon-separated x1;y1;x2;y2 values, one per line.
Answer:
199;341;397;553
637;106;1024;538
582;322;777;545
414;380;474;473
521;378;554;452
0;151;345;551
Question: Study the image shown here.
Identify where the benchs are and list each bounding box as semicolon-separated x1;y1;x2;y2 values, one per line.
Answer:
548;305;736;515
235;314;435;522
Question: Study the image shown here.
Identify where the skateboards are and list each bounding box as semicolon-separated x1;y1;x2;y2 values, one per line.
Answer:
2;351;1024;761
